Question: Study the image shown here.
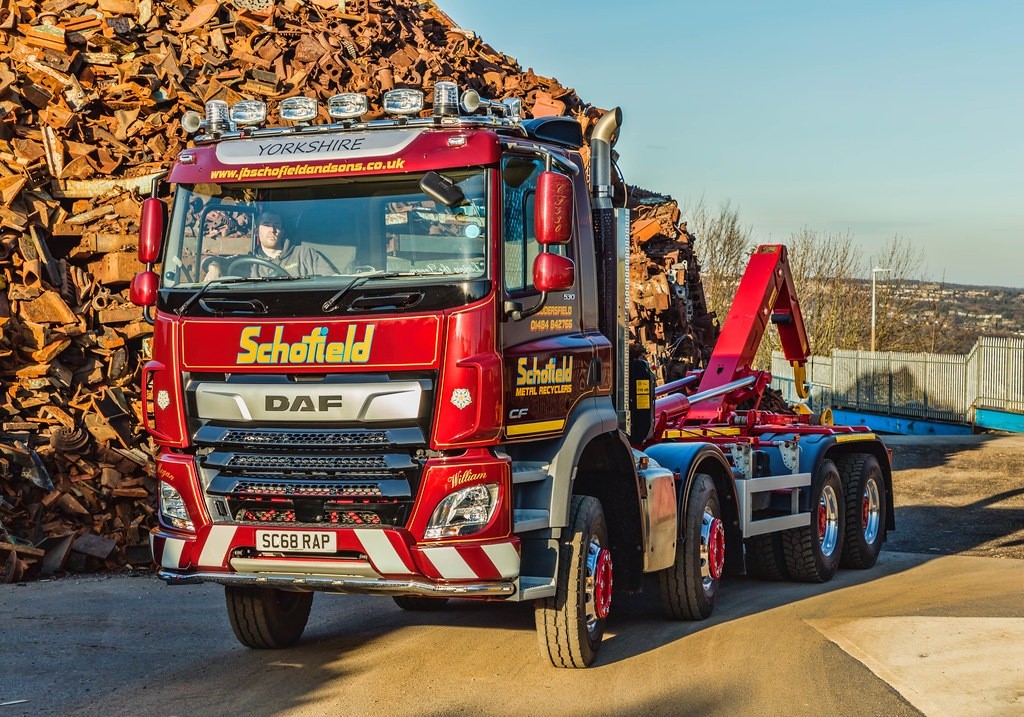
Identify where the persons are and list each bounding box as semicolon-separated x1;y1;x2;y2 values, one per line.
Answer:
201;208;336;282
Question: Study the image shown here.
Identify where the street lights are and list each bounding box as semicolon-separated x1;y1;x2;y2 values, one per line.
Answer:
870;267;891;405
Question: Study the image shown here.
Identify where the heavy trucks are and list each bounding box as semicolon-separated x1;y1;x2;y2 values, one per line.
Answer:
129;83;902;666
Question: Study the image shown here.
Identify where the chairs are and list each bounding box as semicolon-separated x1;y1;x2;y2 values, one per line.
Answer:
296;208;362;274
